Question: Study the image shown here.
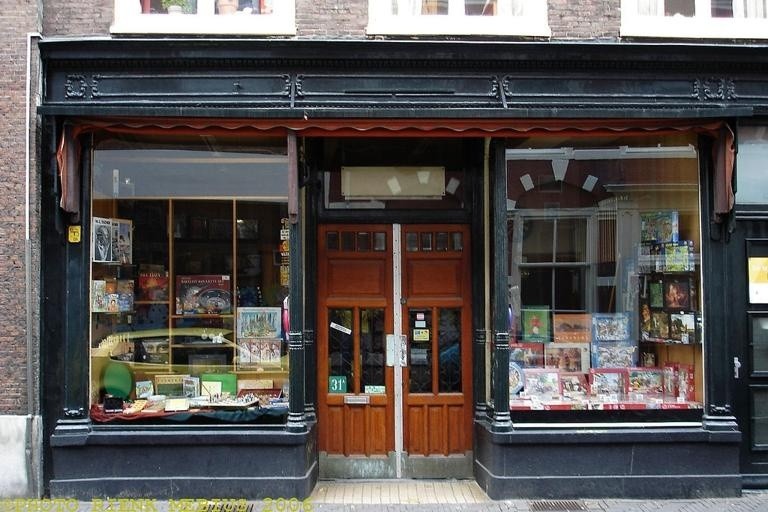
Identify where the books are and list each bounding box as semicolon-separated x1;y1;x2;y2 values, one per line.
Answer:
110;218;132;263
112;220;120;263
94;217;113;262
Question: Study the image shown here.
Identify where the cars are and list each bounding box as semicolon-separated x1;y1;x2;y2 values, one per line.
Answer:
91;327;288;405
